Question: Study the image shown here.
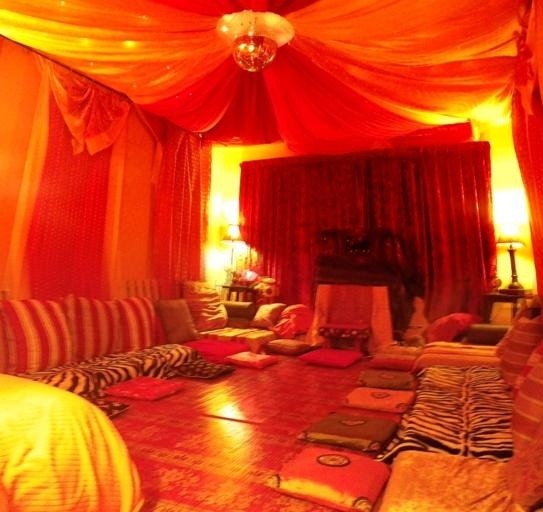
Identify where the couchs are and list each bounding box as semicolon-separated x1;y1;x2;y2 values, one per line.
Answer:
378;297;543;512
0;296;271;397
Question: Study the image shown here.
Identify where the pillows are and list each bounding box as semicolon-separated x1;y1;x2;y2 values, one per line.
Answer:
0;286;226;373
247;302;285;327
494;310;543;447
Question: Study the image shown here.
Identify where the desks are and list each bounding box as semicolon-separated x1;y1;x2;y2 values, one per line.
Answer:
484;293;530;322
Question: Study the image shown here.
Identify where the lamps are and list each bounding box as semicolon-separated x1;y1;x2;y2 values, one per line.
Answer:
215;10;294;72
